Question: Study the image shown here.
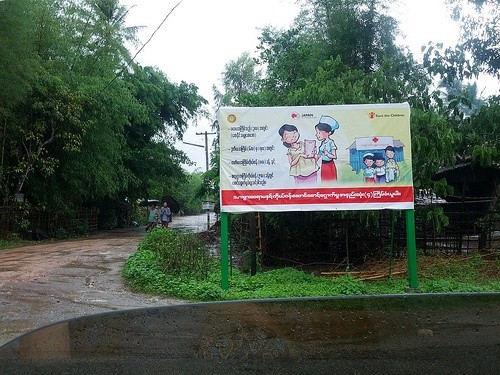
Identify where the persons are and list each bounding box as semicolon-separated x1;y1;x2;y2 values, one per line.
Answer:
145;202;159;234
160;200;171;228
